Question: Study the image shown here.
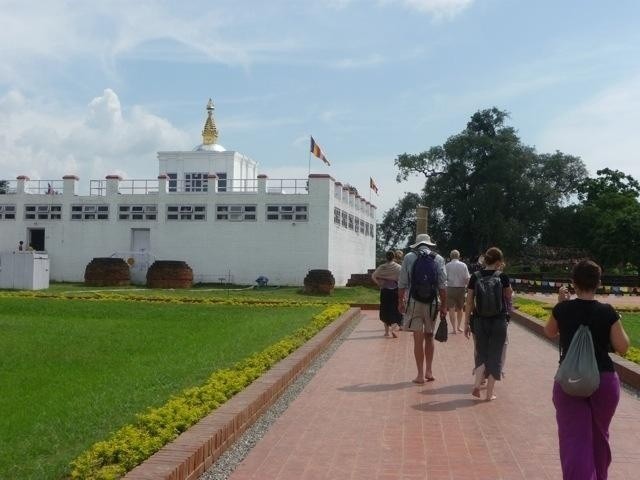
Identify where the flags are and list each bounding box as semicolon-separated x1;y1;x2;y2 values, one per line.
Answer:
310;137;331;168
370;177;379;195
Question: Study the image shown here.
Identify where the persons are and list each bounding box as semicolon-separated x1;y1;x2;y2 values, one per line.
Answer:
540;258;631;479
394;250;404;333
397;233;449;384
443;249;471;335
462;246;515;402
372;248;403;339
473;263;514;390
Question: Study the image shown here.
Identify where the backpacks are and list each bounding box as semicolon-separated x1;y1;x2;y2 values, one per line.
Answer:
473;268;504;319
552;298;601;398
405;250;439;305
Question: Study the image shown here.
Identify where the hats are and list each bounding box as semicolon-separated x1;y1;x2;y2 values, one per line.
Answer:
408;233;438;249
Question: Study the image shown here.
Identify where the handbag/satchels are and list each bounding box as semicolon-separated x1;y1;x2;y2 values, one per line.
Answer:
434;317;448;343
